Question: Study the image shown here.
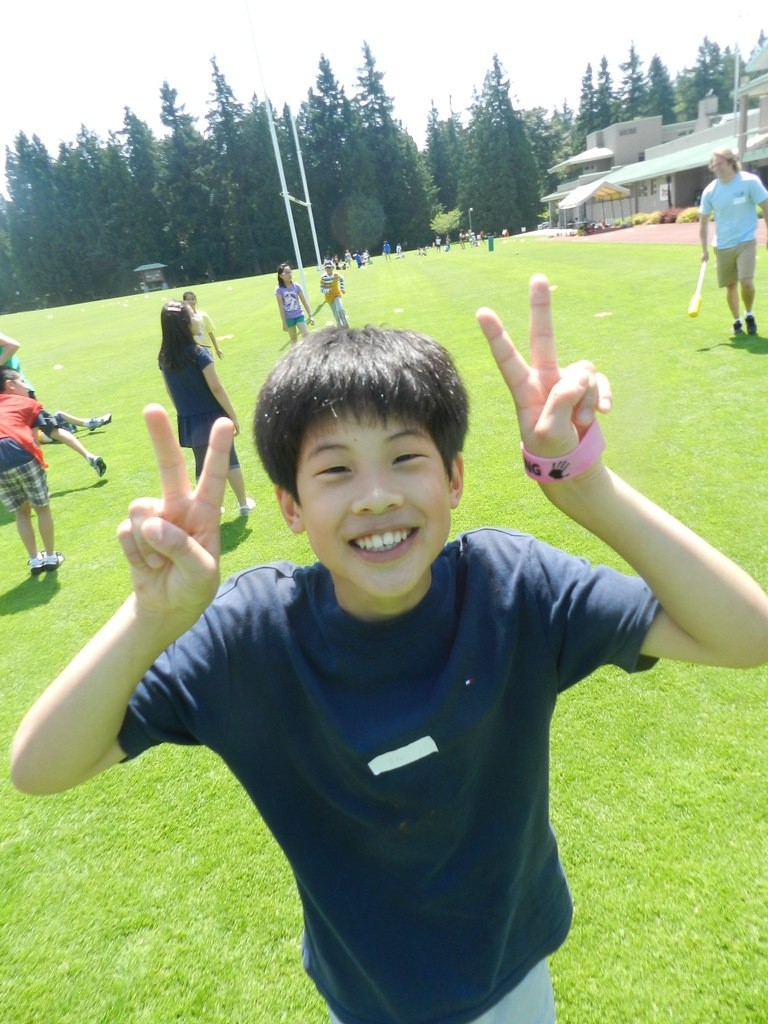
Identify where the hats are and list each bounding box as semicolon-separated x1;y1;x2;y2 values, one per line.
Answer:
324;260;334;268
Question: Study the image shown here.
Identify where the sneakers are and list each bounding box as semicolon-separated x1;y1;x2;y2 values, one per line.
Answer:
734;321;742;332
238;498;256;516
30;554;46;575
90;456;106;477
87;418;104;431
46;552;65;572
220;507;225;513
745;315;756;334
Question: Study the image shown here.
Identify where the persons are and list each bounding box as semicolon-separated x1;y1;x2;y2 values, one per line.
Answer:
383;241;405;263
38;411;112;444
275;263;314;344
0;333;107;478
699;149;768;334
435;229;488;253
8;275;768;1024
183;292;224;360
574;217;584;235
320;260;349;326
324;249;373;269
158;300;256;515
417;246;428;256
0;369;65;575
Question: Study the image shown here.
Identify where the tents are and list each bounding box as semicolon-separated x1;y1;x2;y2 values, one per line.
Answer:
558;180;632;231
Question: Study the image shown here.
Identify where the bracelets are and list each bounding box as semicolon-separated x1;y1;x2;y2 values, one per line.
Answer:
521;418;607;483
